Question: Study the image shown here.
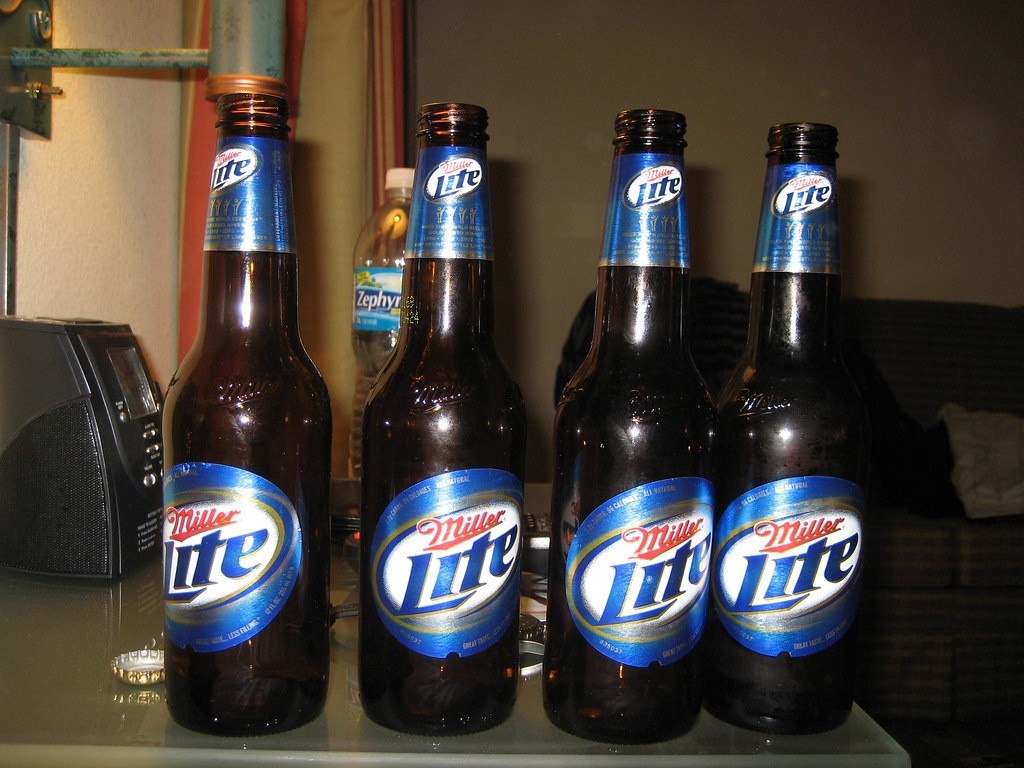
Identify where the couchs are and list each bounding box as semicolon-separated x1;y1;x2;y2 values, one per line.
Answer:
553;269;1024;731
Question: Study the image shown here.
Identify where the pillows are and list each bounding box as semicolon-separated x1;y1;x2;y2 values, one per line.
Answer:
939;401;1024;517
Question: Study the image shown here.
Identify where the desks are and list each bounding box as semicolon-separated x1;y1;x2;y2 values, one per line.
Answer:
0;538;913;768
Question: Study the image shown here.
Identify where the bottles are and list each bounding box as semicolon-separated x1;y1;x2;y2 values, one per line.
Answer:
162;75;332;737
542;111;865;744
347;103;527;737
